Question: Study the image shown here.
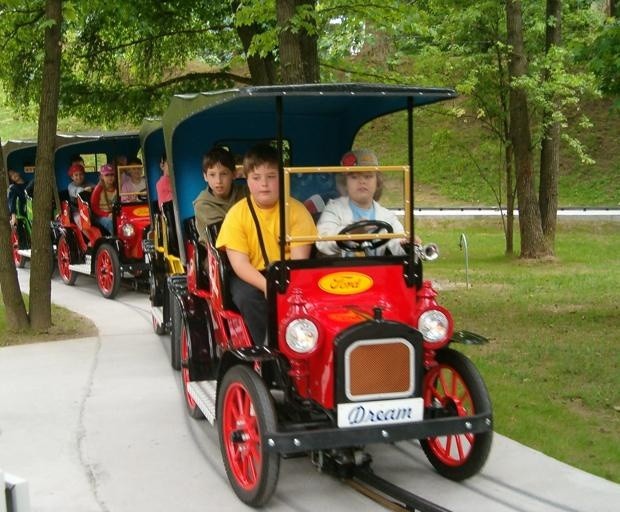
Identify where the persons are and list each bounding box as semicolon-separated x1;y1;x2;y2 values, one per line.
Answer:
191;147;254;246
308;146;423;262
214;144;318;351
4;146;178;259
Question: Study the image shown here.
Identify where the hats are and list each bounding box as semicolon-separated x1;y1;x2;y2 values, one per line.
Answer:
340;150;378;166
68;165;84;176
101;165;115;175
